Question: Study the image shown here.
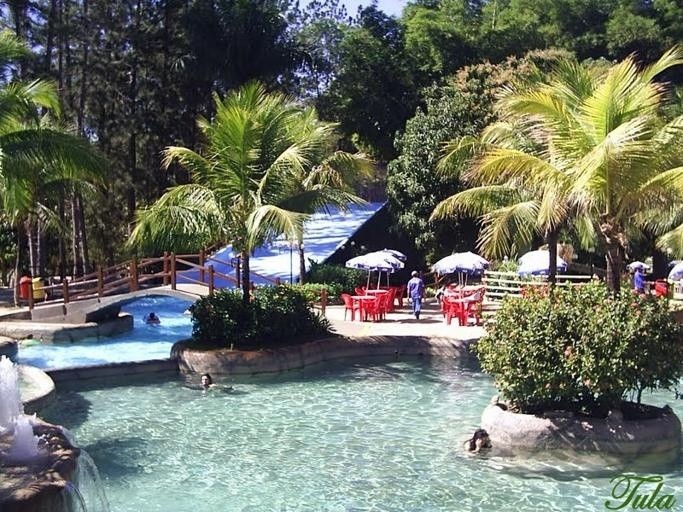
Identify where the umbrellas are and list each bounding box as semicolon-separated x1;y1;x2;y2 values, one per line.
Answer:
432;251;490;287
345;248;407;296
518;250;567;276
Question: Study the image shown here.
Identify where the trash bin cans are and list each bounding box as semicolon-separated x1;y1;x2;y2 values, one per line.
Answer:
18;276;45;306
655;278;674;301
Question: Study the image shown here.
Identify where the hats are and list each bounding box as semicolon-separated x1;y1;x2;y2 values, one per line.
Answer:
411;270;420;277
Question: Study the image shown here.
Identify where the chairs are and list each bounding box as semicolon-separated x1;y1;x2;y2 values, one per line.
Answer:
441;281;485;327
341;282;405;322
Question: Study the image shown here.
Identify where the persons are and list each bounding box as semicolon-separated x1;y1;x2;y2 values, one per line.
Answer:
465;428;492;453
406;271;425;319
146;312;160;324
19;270;51;302
634;265;654;293
201;373;217;391
